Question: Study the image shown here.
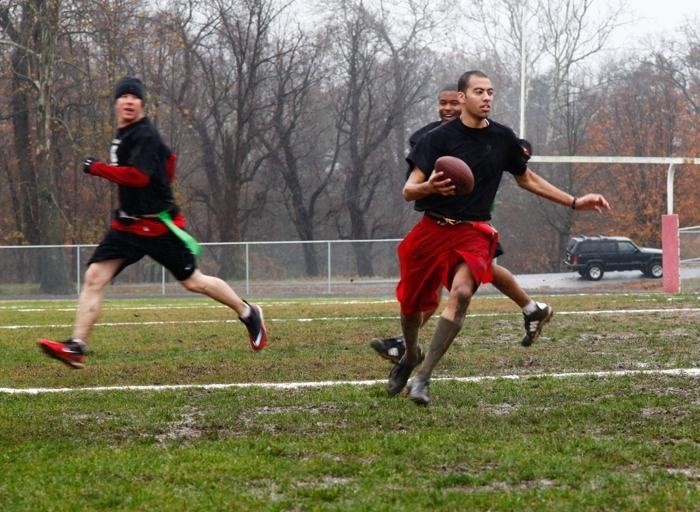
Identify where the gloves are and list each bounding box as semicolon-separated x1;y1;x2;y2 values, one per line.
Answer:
83;157;94;173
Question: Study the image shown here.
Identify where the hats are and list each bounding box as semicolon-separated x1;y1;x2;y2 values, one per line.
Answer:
115;76;143;99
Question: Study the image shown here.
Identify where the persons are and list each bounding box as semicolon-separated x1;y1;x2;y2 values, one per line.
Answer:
34;73;269;370
383;67;615;405
364;82;554;371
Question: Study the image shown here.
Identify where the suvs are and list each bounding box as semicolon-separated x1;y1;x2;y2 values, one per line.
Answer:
562;234;663;282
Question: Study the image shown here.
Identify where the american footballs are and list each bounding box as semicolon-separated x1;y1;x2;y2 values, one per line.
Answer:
434;155;475;196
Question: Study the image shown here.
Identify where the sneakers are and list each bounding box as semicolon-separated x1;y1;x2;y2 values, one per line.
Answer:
371;337;430;403
238;300;266;351
36;338;87;368
522;303;553;347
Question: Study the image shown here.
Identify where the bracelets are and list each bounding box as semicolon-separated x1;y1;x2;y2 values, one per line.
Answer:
570;195;578;211
